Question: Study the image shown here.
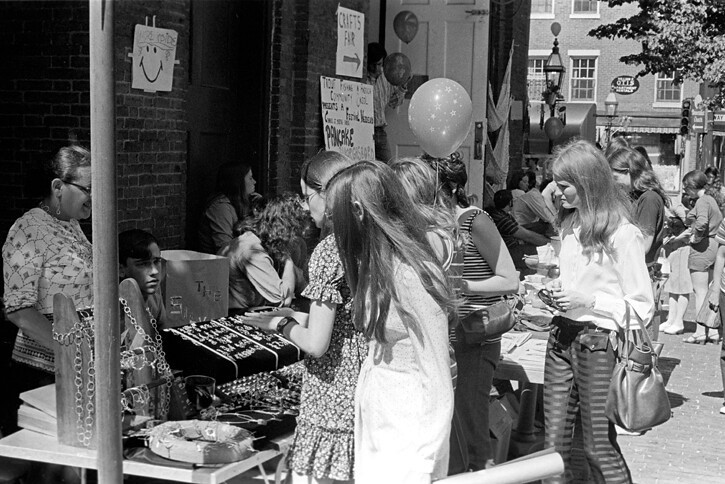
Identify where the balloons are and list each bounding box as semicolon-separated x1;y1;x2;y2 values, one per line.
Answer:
393;11;419;44
408;77;472;158
384;52;412;86
545;117;564;140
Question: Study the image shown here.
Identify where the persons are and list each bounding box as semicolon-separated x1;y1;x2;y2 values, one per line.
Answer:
366;42;413;164
538;140;656;484
118;228;165;415
2;145;95;437
490;134;725;435
240;151;521;484
198;161;311;316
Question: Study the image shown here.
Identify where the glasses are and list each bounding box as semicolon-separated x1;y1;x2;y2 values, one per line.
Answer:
682;184;689;192
61;179;91;195
299;190;319;210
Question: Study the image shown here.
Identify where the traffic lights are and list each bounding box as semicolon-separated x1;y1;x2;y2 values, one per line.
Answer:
679;100;692;135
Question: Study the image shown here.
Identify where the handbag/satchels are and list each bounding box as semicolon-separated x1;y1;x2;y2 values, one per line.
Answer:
454;302;517;347
697;283;722;329
605;301;671;432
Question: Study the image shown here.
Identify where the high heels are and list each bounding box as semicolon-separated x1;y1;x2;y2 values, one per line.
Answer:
658;319;672;331
683;334;706;345
664;323;684;334
706;333;719;345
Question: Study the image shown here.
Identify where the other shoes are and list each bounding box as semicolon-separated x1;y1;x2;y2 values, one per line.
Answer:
720;407;725;414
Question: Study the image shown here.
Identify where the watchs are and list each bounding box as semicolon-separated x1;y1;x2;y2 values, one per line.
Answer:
277;316;299;339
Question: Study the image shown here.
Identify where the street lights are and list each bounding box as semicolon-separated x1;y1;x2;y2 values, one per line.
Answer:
604;85;619;143
543;38;567;155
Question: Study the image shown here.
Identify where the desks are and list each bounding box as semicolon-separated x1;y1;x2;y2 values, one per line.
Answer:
499;332;547;443
0;428;294;484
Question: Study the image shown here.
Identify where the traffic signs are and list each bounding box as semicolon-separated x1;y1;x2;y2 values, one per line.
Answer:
690;110;704;134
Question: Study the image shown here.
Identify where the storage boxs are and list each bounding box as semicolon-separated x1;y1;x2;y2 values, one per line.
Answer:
160;250;229;329
